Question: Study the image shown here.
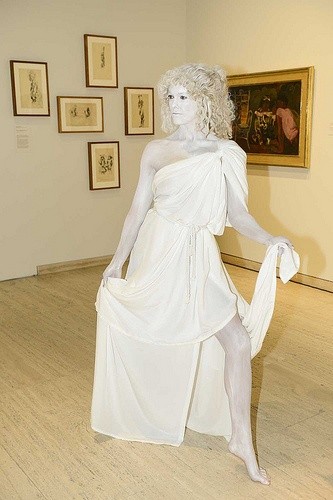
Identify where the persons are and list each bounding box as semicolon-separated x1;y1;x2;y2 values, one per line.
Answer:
88;64;299;484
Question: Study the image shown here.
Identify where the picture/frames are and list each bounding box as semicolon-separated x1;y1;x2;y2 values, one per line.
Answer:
124;87;155;136
226;66;315;169
56;96;104;133
88;141;121;190
9;59;50;117
84;34;118;88
